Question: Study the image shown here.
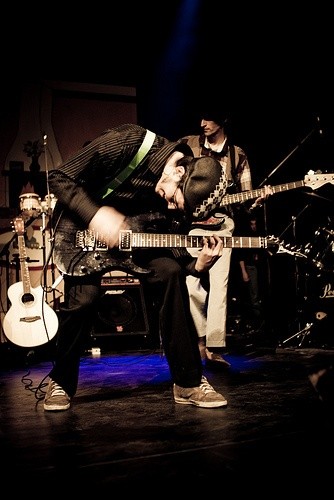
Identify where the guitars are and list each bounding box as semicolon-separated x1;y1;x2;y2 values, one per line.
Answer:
51;211;307;277
192;172;334;222
2;217;59;347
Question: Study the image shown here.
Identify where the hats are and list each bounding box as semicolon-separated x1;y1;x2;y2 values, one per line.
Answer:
183;155;228;221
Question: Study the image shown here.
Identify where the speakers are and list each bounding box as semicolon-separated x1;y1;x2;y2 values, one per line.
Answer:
89;284;163;356
226;263;259;318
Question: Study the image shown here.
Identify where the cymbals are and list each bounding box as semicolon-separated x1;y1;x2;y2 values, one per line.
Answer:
302;191;330;201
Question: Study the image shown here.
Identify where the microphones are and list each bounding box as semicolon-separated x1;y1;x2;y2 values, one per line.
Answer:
316;118;323;135
49;274;64;293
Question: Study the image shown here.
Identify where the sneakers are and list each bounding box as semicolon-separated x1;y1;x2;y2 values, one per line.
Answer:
173;375;227;408
43;378;71;411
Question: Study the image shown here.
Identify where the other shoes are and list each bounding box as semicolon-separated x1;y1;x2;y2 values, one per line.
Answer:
205;348;232;370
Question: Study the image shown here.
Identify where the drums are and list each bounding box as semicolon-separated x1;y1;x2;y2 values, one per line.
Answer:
299;225;334;272
45;193;58;214
20;193;41;213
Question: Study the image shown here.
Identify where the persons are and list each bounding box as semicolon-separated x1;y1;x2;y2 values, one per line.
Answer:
44;123;228;411
177;104;271;369
239;218;260;306
